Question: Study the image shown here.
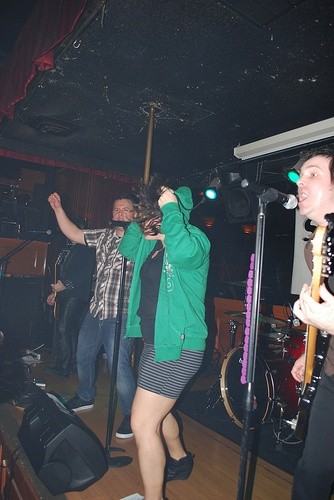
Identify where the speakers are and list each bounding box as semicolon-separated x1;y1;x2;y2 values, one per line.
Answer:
222;184;259;225
18;391;109;496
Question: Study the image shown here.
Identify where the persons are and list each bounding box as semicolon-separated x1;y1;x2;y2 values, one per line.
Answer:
44;223;96;376
291;146;334;500
118;186;211;500
47;189;138;438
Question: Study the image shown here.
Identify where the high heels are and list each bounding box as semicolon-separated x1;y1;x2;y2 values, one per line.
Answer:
163;451;194;482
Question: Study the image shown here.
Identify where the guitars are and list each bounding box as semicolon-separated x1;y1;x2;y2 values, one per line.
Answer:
293;225;334;442
53;252;64;318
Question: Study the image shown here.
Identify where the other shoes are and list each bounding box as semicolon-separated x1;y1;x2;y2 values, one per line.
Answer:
48;367;69;379
68;364;78;374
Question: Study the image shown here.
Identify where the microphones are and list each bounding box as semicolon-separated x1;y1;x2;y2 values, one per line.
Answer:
289;302;301;326
241;178;298;209
29;229;52;235
108;219;130;227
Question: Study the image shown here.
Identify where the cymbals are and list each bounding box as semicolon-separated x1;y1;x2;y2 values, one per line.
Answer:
224;310;287;324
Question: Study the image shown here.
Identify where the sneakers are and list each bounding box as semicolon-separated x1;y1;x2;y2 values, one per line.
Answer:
66;395;94;412
115;414;134;439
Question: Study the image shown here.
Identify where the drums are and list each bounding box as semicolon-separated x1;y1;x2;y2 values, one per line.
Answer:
221;348;301;430
285;330;306;358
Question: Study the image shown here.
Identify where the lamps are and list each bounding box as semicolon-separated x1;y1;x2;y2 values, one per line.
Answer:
205;177;220;201
232;117;334;161
283;152;310;183
